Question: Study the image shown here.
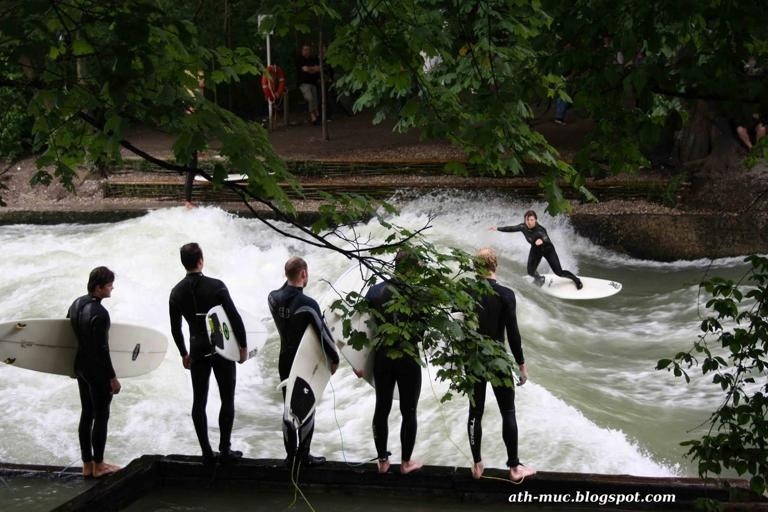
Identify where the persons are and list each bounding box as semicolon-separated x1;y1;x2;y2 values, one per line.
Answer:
169;243;247;464
298;45;321;124
554;71;567;126
66;266;121;478
267;256;339;464
451;248;527;480
364;249;425;474
488;209;583;290
737;114;766;150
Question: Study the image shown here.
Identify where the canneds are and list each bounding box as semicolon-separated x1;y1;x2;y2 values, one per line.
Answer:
261;64;285;102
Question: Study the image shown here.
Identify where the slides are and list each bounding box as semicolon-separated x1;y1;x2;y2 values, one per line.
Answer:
205;305;269;362
423;312;467;375
523;274;623;300
277;253;401;431
0;318;169;379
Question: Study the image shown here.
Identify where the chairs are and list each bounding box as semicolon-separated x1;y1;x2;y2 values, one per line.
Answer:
574;278;582;289
202;451;243;462
283;454;326;465
